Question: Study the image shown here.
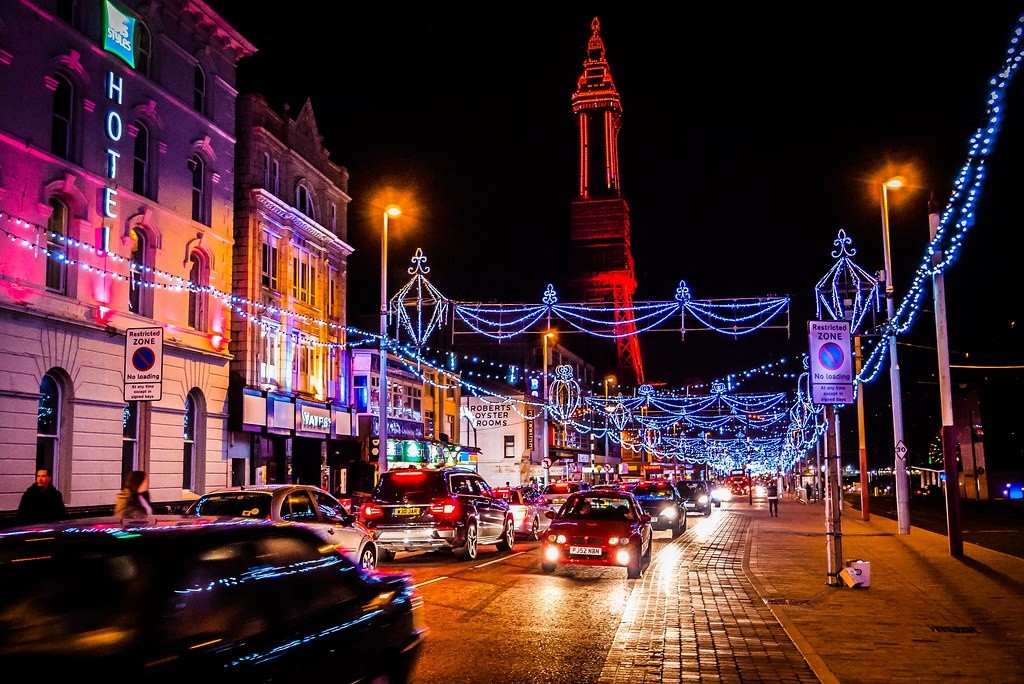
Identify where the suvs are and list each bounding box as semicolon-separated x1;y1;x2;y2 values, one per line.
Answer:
361;468;517;562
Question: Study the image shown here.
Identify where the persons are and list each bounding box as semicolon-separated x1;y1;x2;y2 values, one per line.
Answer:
573;501;592;519
835;482;841;516
16;467;68;516
816;478;825;500
766;480;780;517
114;471;156;516
625;504;635;519
805;482;812;501
506;481;510;487
624;435;765;535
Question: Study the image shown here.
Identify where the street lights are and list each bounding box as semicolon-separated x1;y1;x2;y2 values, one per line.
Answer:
880;173;915;537
705;432;711;481
672;422;679;480
542;330;559;485
603;377;616;484
641;405;648;469
378;202;408;473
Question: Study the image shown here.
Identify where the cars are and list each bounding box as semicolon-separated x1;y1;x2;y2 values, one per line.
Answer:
182;482;380;576
0;515;429;684
676;479;711;516
629;480;688;539
709;468;750;509
539;490;653;580
542;481;584;516
490;484;557;539
